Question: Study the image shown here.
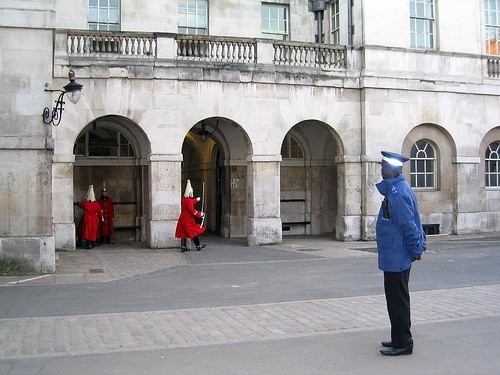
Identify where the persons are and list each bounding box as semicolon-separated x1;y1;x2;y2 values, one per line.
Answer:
97;181;115;243
376;151;428;356
74;184;103;249
175;179;207;252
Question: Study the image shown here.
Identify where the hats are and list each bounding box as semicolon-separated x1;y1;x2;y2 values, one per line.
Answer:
184;179;194;198
86;184;96;202
377;151;409;168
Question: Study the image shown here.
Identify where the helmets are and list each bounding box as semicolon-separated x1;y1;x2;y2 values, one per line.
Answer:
101;181;107;191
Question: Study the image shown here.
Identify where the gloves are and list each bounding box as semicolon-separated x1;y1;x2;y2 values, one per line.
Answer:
196;197;200;201
200;212;203;217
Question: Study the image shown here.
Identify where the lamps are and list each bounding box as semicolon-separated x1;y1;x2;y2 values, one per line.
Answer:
191;119;219;143
41;70;83;126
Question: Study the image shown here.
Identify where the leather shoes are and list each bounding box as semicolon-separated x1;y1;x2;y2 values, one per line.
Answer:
182;247;190;252
382;338;413;346
380;344;413;355
197;243;206;251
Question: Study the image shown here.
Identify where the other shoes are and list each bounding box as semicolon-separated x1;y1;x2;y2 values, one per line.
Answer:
78;242;81;246
88;243;95;248
